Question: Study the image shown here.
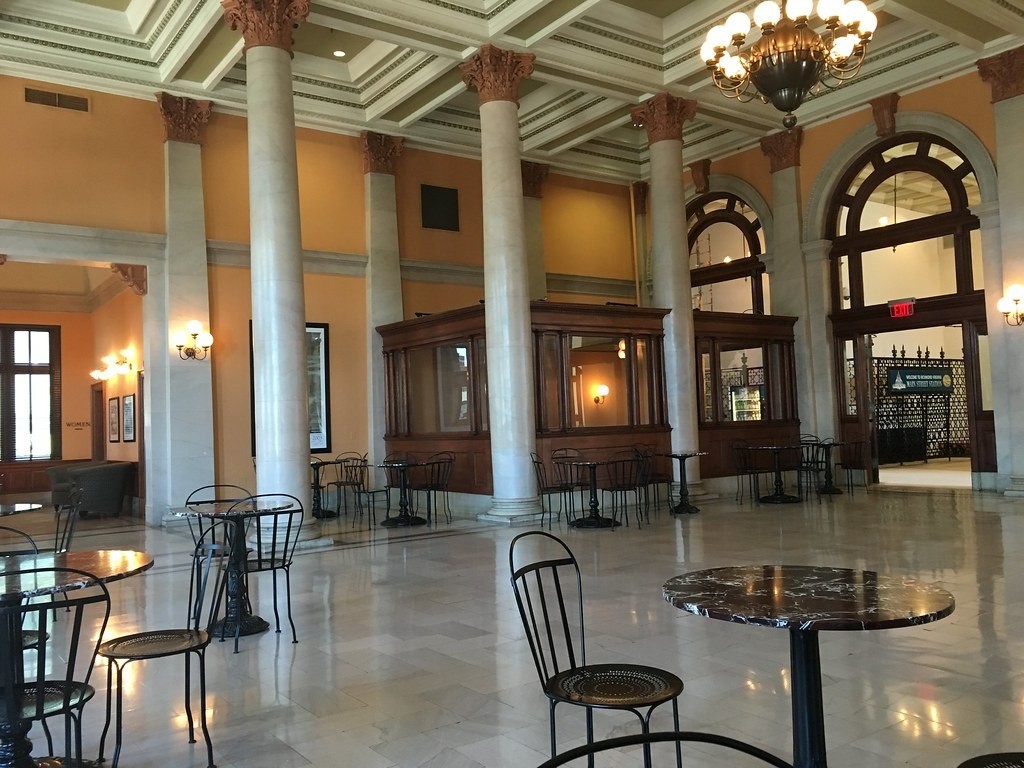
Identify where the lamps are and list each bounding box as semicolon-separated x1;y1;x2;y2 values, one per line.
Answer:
617;338;626;360
997;284;1024;326
701;1;877;128
595;384;610;406
176;322;214;360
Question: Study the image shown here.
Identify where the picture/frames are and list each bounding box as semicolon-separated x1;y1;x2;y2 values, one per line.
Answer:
108;397;120;443
123;393;135;442
249;319;333;458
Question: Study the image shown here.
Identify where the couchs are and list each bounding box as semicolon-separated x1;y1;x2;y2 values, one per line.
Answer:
48;460;133;520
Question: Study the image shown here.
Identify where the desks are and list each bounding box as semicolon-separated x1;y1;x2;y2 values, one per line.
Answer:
662;565;954;768
169;501;294;636
655;451;710;514
0;550;153;768
1;502;42;556
736;446;805;503
375;459;453;527
310;459;349;520
797;440;865;494
553;457;642;528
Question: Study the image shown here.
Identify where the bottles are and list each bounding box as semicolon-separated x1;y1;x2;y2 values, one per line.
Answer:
737;411;761;421
736;401;760;409
735;390;759;399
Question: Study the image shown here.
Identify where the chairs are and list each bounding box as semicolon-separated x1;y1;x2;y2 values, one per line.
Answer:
731;432;872;504
412;451;457;527
600;443;676;531
344;456;388;531
508;531;684;768
310;451;367;519
16;488;83;623
1;525;111;768
529;447;588;531
95;519;239;768
383;453;417;522
